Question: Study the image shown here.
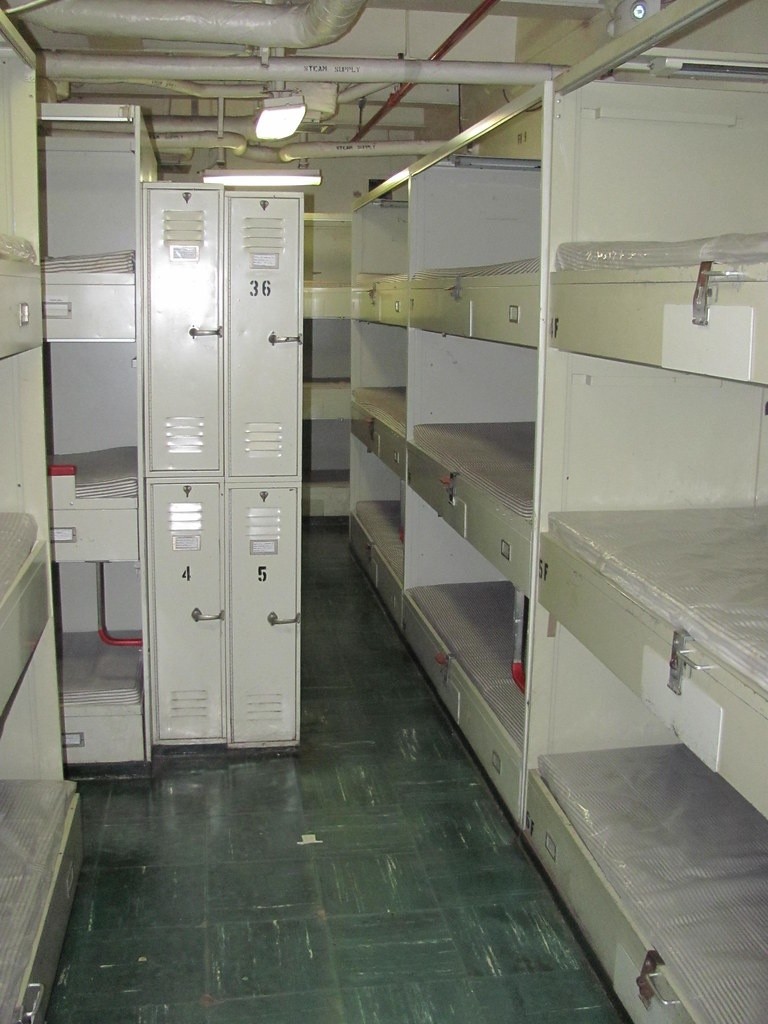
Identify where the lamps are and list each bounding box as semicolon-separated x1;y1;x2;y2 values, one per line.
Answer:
254;89;307;142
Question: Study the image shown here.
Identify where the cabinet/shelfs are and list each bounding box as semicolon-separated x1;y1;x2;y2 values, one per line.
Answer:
0;0;768;1024
